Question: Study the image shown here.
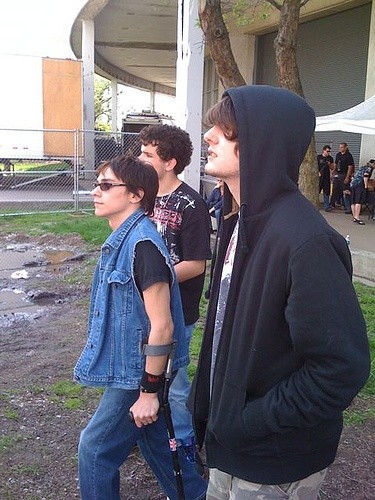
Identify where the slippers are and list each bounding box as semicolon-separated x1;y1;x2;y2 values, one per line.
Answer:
354;217;365;225
354;218;357;222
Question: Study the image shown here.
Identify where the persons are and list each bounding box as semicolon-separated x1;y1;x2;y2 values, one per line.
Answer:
349;159;375;225
317;145;334;211
329;160;345;209
138;124;212;478
331;142;355;213
186;85;373;500
207;178;224;233
74;156;209;499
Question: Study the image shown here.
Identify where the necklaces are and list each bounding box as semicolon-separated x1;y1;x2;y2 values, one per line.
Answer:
156;180;182;208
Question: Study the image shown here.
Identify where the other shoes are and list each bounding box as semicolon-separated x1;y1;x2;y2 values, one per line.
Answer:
326;206;334;212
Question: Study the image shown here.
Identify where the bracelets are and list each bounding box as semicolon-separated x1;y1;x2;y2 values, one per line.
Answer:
140;370;166;393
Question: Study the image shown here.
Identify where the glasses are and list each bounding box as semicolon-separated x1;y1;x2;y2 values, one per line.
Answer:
324;149;331;153
92;180;126;190
